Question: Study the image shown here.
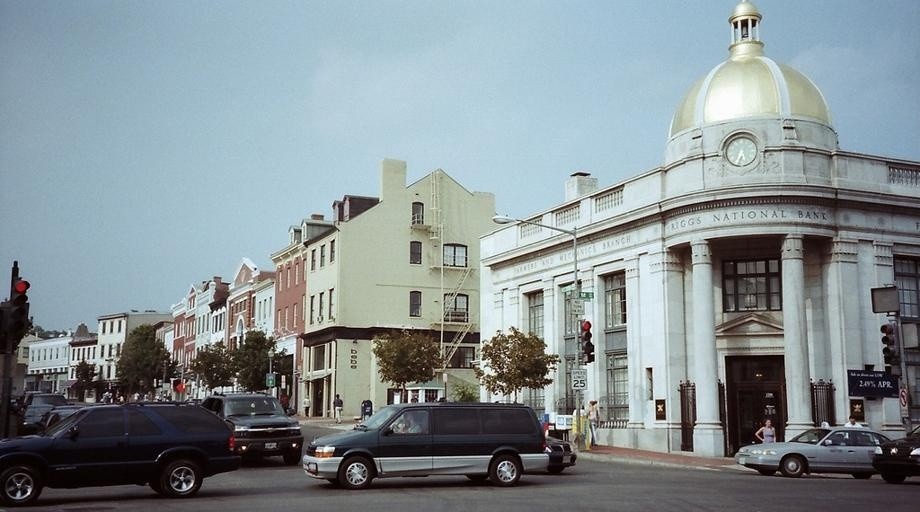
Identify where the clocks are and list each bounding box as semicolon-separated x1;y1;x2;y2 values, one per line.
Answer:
725;135;758;166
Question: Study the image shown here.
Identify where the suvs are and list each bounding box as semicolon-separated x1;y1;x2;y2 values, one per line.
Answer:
1;400;245;507
203;390;304;469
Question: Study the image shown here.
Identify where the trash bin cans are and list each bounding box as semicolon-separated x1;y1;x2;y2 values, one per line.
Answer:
548;423;572;442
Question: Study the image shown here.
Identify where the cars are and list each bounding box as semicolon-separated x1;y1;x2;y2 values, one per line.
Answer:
873;424;920;484
734;425;892;481
542;436;575;475
19;391;85;436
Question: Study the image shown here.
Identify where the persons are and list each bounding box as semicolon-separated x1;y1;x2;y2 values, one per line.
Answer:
755;418;777;446
844;413;863;428
333;393;344;424
99;390;172;405
303;394;311;419
572;399;600;451
818;421;831;446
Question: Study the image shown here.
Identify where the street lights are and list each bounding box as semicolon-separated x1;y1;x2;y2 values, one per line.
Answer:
267;348;275;394
176;363;184;399
493;214;586;451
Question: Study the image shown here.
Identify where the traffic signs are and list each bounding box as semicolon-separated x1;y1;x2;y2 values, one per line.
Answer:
571;370;587;390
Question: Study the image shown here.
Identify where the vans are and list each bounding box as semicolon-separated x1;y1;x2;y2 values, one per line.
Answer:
305;399;549;492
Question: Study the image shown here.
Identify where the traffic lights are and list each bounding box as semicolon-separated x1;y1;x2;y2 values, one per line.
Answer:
583;345;595;363
885;364;901;378
580;319;593;351
9;278;30;333
880;323;900;366
869;286;901;314
